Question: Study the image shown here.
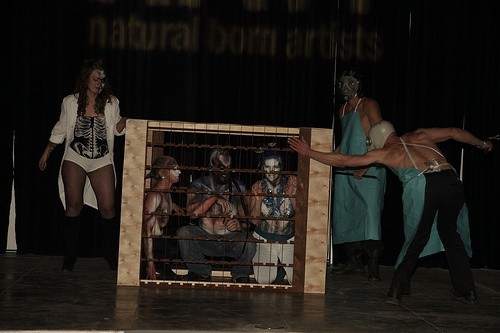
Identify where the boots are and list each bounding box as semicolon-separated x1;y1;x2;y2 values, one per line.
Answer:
62;216;79;272
367;249;379;280
101;216;118;270
331;250;366;276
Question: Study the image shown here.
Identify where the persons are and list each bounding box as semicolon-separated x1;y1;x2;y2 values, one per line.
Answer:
38;57;130;273
176;150;256;284
145;157;187;282
246;151;297;285
286;118;494;306
334;63;383;281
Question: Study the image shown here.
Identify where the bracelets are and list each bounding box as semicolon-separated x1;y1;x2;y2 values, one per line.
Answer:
476;139;485;149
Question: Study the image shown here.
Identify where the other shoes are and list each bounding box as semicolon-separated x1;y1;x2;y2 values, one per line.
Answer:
453;289;477;303
188;271;210;280
274;266;286;283
385;287;402;305
233;277;250;283
